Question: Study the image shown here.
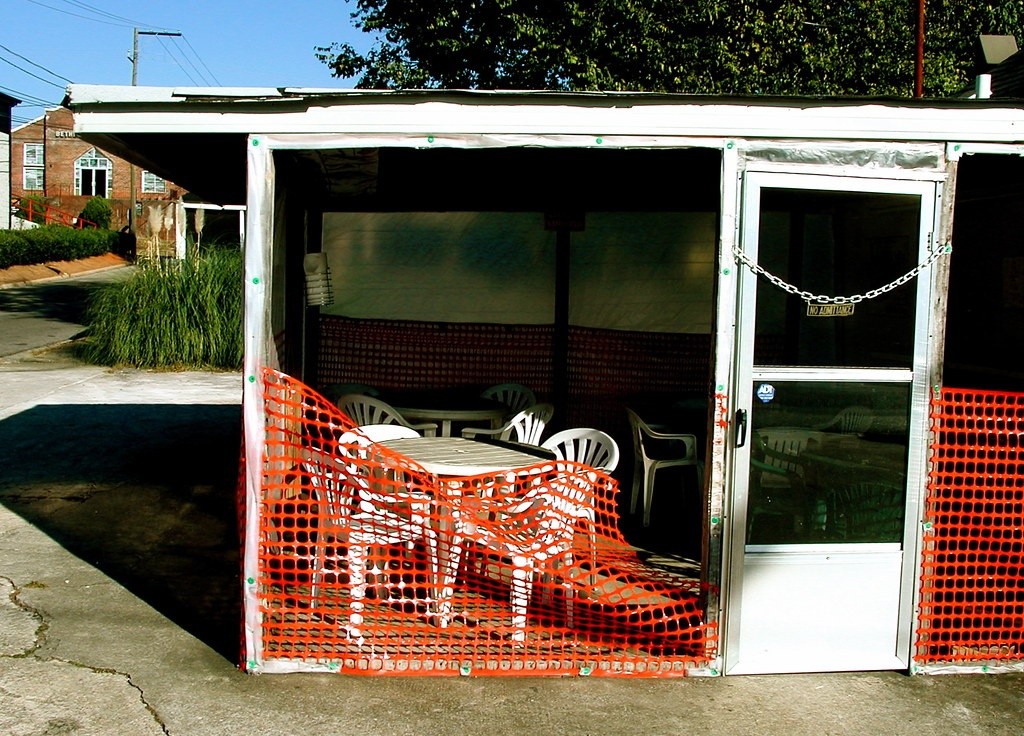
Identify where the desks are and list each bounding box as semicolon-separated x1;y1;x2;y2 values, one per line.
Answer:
392;405;506;440
756;427;904;543
372;437;556;603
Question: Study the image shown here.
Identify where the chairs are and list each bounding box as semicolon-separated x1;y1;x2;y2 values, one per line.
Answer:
329;384;874;648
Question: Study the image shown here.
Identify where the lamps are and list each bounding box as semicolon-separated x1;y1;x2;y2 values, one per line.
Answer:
302;252;333;306
